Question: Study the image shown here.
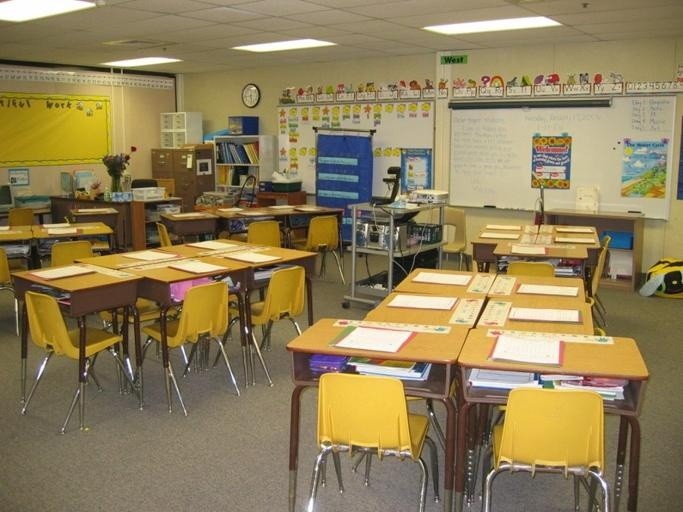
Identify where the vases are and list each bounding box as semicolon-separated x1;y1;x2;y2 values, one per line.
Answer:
111;175;121;192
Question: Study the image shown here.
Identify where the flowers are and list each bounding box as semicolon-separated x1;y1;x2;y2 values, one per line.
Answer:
102;146;136;174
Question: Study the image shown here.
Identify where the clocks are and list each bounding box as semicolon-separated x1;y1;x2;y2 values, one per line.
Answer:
242;84;261;109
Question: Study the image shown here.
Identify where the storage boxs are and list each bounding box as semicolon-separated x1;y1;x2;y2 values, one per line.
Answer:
228;116;259;136
272;180;303;192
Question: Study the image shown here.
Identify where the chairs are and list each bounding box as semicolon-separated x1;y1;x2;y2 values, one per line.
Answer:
8;207;35;226
293;216;346;286
84;298;191;394
584;246;608;329
479;388;610;512
130;179;157;189
307;373;439;512
130;282;241;417
213;267;306;387
155;222;174;246
0;247;23;336
21;291;145;435
430;207;467;271
50;240;94;270
590;235;612;314
247;220;281;249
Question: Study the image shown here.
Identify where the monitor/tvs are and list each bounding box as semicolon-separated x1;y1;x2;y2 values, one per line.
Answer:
0;183;15;212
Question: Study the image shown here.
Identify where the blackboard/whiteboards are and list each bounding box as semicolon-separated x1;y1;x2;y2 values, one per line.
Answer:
448;94;676;222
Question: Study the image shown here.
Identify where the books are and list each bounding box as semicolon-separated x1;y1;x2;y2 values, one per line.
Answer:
553;378;628;401
540;375;583;381
468;368;543;391
254;264;293;280
218;165;255;187
345;357;432;381
553;265;582;276
215;141;259;164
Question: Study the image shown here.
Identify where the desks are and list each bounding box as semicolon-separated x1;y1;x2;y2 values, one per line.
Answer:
158;237;265;371
65;207;121;253
1;226;32;231
118;257;250;414
207;205;287;248
205;245;319;386
363;292;488;330
74;250;207;395
32;222;106;231
471;232;537;273
287;204;345;277
539;225;598;236
536;233;602;275
456;328;650;511
0;232;33;270
10;263;144;432
475;297;595;448
493;244;588;278
487;273;586;303
478;224;539;234
160;211;220;244
394;267;499;298
285;317;469;512
50;194;184;252
33;226;114;270
0;206;52;225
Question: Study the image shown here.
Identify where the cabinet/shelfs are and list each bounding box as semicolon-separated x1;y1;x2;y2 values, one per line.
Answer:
160;111;204;148
150;147;216;213
256;192;307;206
543;208;645;295
213;136;277;194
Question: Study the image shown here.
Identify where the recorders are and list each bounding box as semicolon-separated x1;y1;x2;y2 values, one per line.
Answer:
359;223;407;251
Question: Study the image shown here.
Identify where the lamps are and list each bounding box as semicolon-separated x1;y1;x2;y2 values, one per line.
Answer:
0;1;96;23
100;56;184;68
229;37;338;53
420;16;564;37
